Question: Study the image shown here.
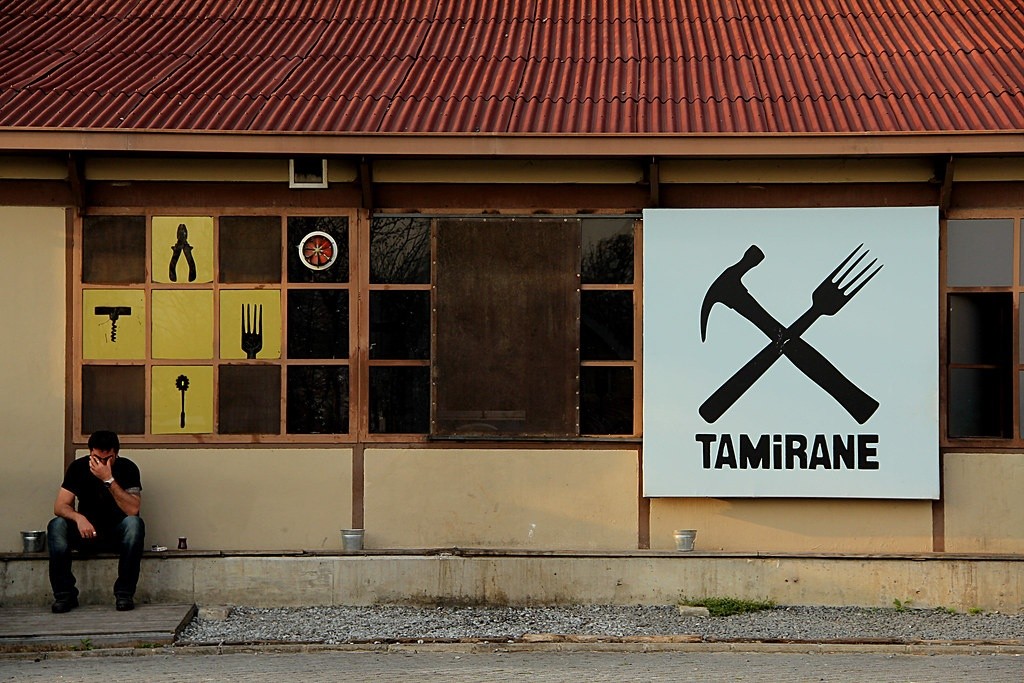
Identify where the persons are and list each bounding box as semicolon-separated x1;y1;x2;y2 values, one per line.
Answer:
47;430;145;613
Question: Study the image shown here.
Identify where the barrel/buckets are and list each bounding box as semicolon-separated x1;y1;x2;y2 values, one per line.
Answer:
673;529;697;552
340;529;365;550
20;530;46;553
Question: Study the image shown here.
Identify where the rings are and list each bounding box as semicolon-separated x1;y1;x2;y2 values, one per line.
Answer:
93;532;97;535
97;460;100;463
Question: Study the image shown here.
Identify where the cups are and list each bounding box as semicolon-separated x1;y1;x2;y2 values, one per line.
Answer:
178;537;187;550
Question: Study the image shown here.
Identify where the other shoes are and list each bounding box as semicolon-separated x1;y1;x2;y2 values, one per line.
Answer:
52;597;78;613
116;600;135;611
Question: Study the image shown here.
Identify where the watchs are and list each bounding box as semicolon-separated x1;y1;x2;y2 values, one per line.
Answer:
104;477;115;488
